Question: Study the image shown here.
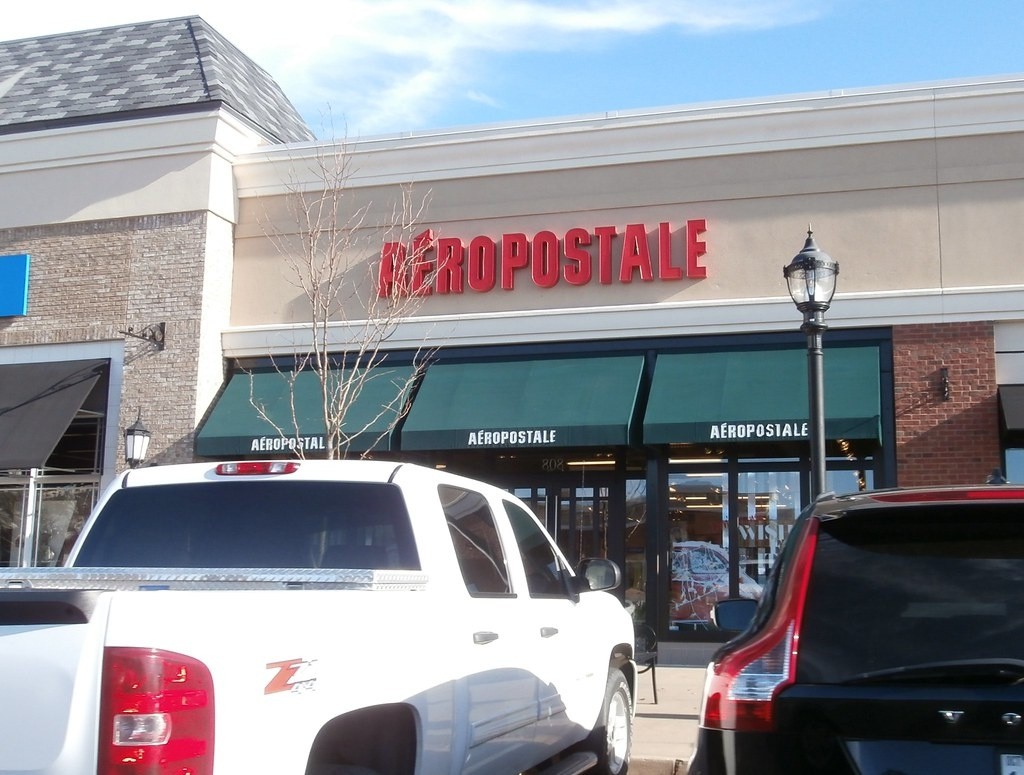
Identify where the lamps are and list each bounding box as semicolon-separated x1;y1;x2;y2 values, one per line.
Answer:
123;406;158;468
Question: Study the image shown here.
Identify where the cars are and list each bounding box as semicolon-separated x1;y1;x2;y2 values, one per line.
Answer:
669;540;764;641
688;470;1024;775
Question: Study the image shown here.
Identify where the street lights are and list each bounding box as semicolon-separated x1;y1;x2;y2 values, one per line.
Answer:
781;218;841;502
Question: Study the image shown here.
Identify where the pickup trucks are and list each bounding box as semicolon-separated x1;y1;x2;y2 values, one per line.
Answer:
0;460;640;775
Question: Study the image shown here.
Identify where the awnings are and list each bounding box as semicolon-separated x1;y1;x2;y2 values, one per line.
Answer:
0;359;110;474
197;366;415;455
401;354;645;450
998;384;1024;429
643;347;882;445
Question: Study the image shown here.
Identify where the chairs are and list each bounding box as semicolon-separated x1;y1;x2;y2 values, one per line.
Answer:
320;546;389;569
633;624;658;705
